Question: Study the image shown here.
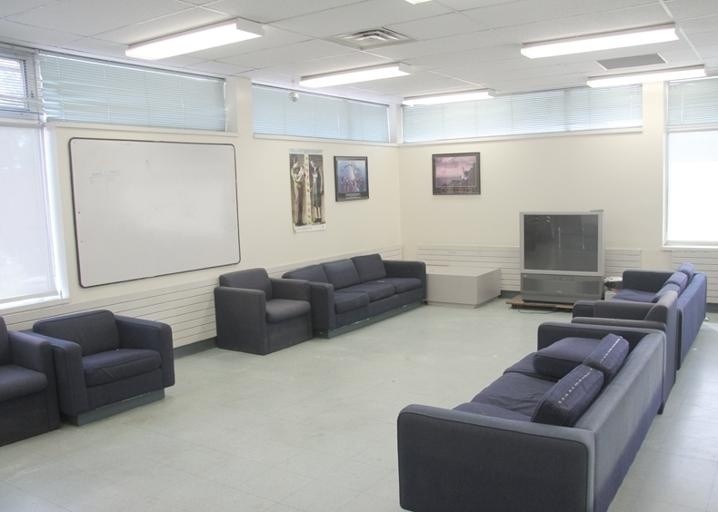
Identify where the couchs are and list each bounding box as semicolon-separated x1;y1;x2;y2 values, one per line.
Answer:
19;309;176;427
570;261;708;371
396;320;669;512
212;266;314;357
569;290;678;416
0;315;63;446
281;253;427;339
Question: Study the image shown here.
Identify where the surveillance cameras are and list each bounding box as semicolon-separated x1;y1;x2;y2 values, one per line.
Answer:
289;92;299;102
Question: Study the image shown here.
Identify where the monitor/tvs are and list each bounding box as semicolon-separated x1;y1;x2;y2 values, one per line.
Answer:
520;210;605;276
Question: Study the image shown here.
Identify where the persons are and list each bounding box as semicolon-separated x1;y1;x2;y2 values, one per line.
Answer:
340;175;366;194
309;159;325;226
290;159;303;226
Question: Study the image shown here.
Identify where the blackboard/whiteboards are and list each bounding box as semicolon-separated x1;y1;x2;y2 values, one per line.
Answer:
68;137;241;289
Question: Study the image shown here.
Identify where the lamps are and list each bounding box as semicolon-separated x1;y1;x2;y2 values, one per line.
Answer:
401;87;498;108
518;22;680;60
585;63;711;90
124;16;265;60
298;61;412;89
